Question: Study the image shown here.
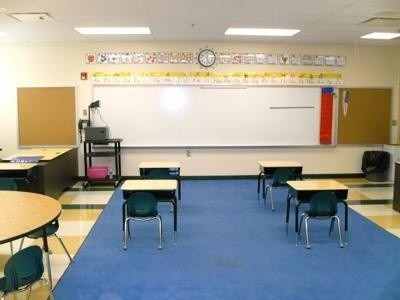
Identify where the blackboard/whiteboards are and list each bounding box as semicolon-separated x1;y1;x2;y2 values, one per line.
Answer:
91;84;339;148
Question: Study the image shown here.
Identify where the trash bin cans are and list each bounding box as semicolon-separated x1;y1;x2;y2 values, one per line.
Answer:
364;151;390;182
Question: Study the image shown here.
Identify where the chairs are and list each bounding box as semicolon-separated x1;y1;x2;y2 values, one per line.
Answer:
123;192;163;249
296;189;346;246
264;167;297;211
0;245;55;300
19;221;74;286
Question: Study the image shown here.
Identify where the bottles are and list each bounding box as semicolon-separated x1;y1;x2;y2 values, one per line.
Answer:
109;169;113;179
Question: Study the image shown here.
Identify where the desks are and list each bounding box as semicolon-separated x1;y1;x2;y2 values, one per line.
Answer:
139;162;182;200
0;189;62;280
285;180;349;240
257;161;303;204
121;179;178;230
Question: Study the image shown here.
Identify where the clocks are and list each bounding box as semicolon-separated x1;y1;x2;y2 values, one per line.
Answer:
198;49;216;67
